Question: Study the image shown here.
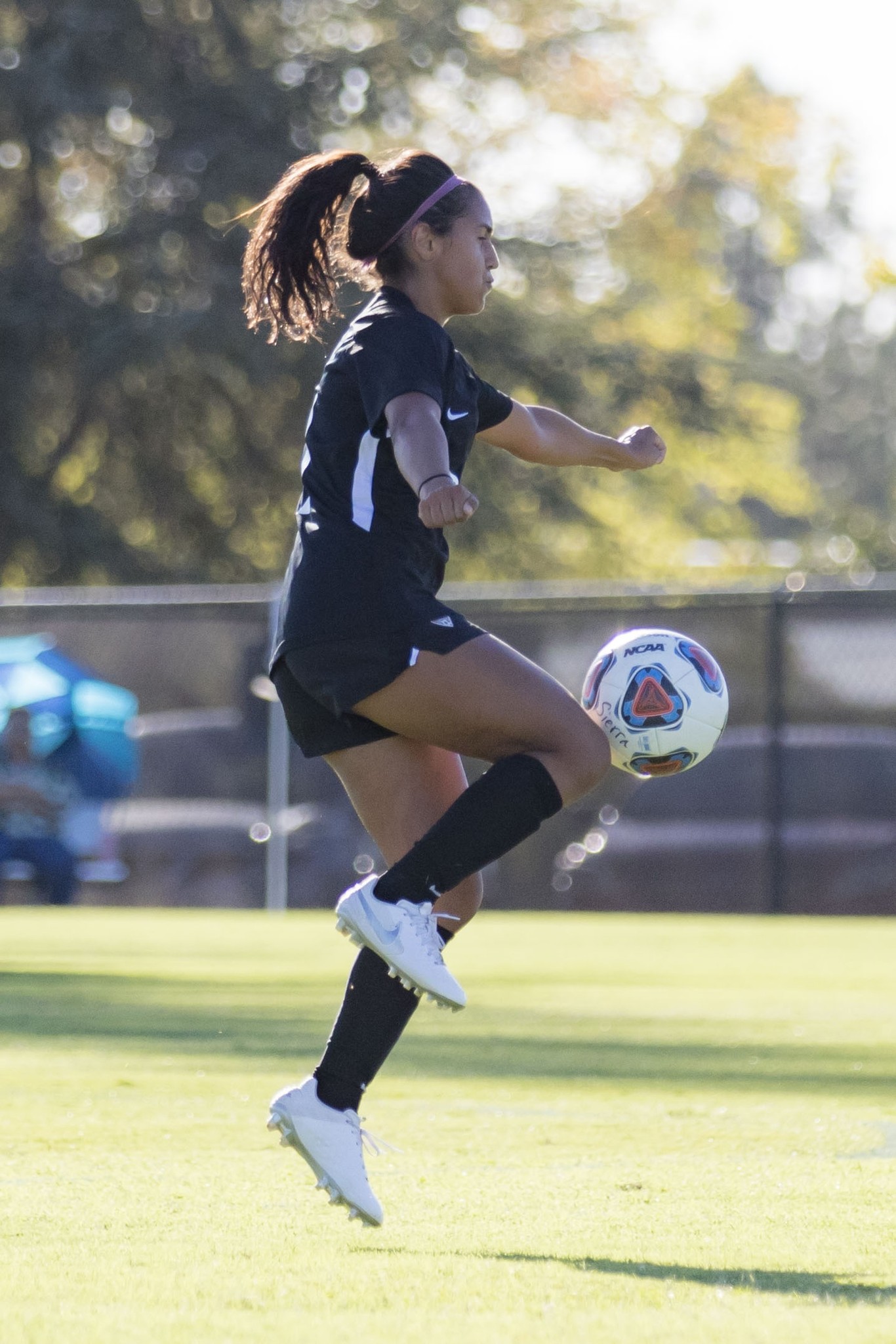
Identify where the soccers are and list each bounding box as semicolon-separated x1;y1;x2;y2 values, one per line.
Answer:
583;626;732;782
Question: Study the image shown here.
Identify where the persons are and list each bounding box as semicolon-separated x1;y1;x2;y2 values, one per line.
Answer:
213;152;666;1234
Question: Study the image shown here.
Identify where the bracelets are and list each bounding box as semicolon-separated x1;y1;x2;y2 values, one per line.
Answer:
418;474;456;502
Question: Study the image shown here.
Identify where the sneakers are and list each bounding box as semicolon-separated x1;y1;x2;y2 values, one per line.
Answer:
336;874;467;1013
265;1078;389;1229
